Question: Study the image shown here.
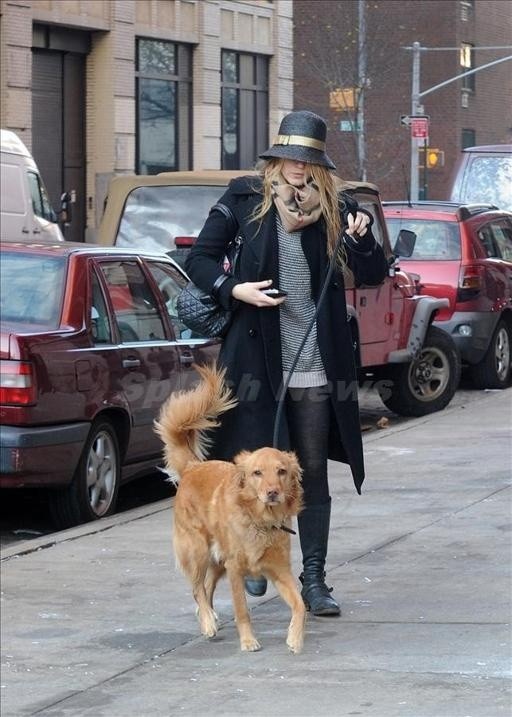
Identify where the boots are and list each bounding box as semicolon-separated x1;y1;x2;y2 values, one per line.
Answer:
244;575;267;597
300;505;340;615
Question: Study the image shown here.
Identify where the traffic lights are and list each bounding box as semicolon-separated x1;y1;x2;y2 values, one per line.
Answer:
423;147;446;167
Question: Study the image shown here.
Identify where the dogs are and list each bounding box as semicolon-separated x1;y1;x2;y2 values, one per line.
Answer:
150;360;310;654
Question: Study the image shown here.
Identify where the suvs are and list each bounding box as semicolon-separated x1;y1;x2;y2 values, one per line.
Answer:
447;142;512;211
93;168;463;416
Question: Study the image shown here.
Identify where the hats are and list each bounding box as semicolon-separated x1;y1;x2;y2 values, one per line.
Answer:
259;111;337;169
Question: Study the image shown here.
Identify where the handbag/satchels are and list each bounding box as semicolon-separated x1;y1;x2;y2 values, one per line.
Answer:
177;286;232;338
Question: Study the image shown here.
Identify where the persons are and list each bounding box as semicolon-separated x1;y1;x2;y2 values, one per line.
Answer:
184;135;389;616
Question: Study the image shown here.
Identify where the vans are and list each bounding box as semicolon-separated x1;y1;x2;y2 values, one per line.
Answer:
0;126;73;241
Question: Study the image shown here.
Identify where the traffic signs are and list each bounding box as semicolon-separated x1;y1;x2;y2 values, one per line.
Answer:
399;113;430;125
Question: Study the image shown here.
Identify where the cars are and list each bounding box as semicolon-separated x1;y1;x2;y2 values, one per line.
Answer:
0;240;226;527
377;200;512;391
